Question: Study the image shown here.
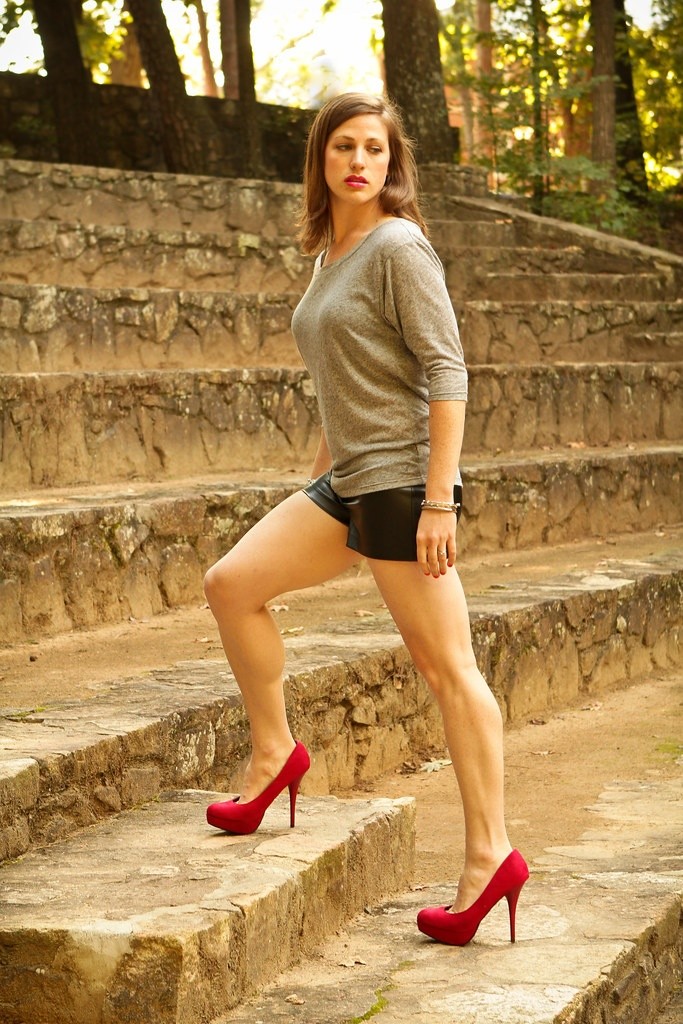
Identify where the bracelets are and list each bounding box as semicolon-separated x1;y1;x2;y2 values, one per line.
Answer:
420;500;458;513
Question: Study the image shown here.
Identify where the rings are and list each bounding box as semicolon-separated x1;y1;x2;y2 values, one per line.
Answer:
437;550;446;555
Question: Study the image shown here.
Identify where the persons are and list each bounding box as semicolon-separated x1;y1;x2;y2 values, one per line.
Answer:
203;93;528;946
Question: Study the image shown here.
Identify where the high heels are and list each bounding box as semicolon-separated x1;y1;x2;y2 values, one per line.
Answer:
207;740;310;835
417;848;529;946
